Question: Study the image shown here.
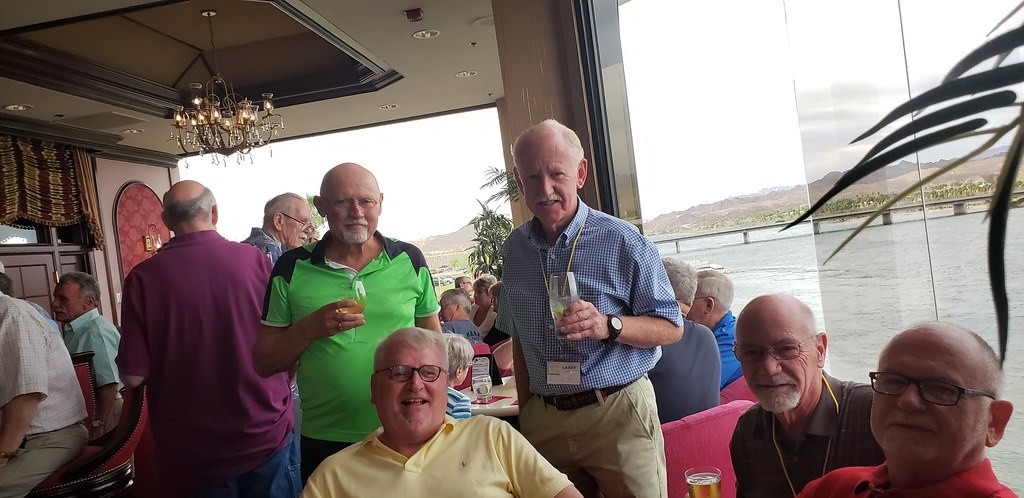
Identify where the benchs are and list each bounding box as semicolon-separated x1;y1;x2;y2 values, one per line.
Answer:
660;399;756;498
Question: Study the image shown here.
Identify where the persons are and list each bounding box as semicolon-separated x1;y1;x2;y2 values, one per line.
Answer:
0;260;124;498
241;192;314;435
686;294;886;498
438;274;511;418
250;163;442;488
494;119;683;498
299;327;584;498
115;180;298;498
649;257;744;424
797;322;1019;498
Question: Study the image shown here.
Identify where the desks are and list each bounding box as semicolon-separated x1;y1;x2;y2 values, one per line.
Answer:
459;375;520;417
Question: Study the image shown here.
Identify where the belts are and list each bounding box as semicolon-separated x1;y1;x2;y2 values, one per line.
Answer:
537;375;644;411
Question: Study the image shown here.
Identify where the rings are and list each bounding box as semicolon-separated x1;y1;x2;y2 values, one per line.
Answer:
337;322;343;332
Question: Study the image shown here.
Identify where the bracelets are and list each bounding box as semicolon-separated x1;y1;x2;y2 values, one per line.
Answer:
0;452;14;458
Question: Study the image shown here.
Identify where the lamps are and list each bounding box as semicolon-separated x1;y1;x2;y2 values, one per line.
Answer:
143;223;163;256
166;11;284;170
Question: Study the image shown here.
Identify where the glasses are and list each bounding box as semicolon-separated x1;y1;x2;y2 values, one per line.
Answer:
375;365;448;383
869;372;997;407
732;337;812;364
463;281;472;286
332;198;382;210
281;212;314;234
469;361;474;367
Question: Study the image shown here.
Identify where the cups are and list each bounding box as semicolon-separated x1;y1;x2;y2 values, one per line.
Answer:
471;375;493;403
549;272;580;340
337;280;366;315
684;466;722;498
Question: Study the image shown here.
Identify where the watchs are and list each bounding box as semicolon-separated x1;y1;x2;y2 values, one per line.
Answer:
605;313;623;342
92;419;104;428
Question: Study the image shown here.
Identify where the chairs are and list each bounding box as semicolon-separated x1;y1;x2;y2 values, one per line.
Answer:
454;343;491;392
71;351;98;440
490;337;514;379
25;355;147;498
